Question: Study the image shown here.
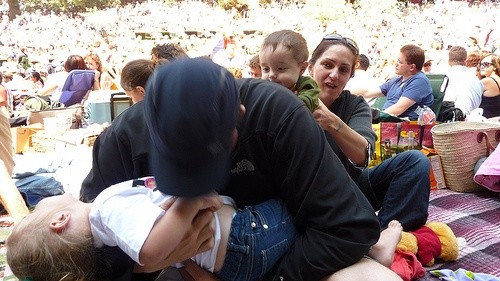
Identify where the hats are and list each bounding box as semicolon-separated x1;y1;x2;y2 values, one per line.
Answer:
144;58;239;197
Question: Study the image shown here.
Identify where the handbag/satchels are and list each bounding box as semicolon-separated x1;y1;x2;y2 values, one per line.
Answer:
430;122;500;192
437;101;465;124
368;120;438;167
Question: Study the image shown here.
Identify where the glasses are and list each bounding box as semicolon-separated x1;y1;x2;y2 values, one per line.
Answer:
312;33;359;64
478;62;495;67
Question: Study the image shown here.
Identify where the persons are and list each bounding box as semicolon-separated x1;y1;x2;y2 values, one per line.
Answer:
0;72;44;107
249;30;434;231
79;58;403;281
422;46;500;119
6;176;403;281
120;43;189;104
41;54;118;107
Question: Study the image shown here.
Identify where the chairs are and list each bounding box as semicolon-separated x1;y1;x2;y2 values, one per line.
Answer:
368;73;449;124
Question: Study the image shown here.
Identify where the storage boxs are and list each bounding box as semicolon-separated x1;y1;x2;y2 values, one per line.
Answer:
88;90;132;124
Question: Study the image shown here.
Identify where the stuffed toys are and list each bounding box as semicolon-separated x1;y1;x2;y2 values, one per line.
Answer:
396;222;459;266
